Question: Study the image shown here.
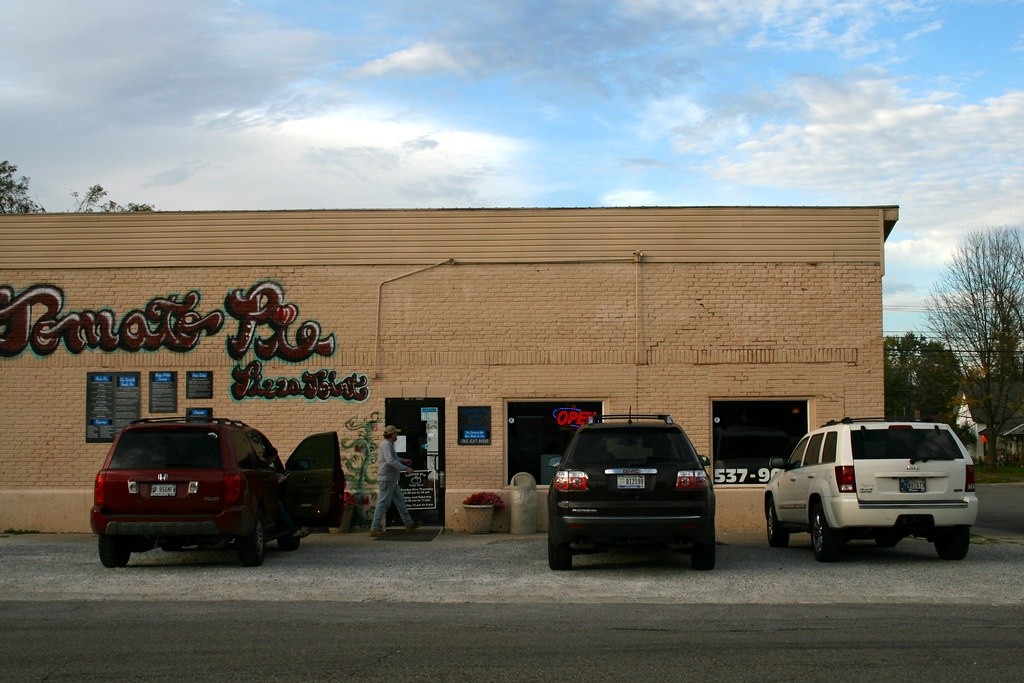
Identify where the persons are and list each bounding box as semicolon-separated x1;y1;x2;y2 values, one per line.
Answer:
369;426;421;537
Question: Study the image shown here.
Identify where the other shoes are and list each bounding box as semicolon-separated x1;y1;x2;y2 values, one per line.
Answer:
406;520;422;533
369;530;388;537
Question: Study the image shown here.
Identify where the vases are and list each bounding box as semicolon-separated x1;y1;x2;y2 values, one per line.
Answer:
462;504;493;534
328;504;353;534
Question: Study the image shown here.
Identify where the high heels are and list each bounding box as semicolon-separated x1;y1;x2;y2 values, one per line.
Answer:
291;531;309;543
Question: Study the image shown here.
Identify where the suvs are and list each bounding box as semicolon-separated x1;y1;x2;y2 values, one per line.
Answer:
89;415;348;568
545;413;717;570
763;415;980;560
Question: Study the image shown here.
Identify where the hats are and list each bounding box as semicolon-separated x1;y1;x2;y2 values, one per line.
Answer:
384;426;401;435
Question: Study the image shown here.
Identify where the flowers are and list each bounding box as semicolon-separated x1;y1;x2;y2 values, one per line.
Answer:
342;493;357;505
463;492;506;511
